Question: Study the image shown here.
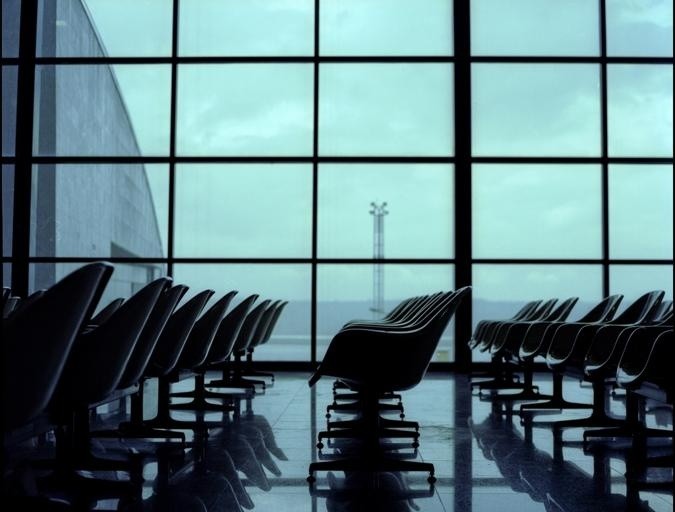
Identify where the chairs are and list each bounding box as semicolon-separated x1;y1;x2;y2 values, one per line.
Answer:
323;391;423;512
307;285;474;484
469;289;675;450
466;385;674;512
0;262;288;511
